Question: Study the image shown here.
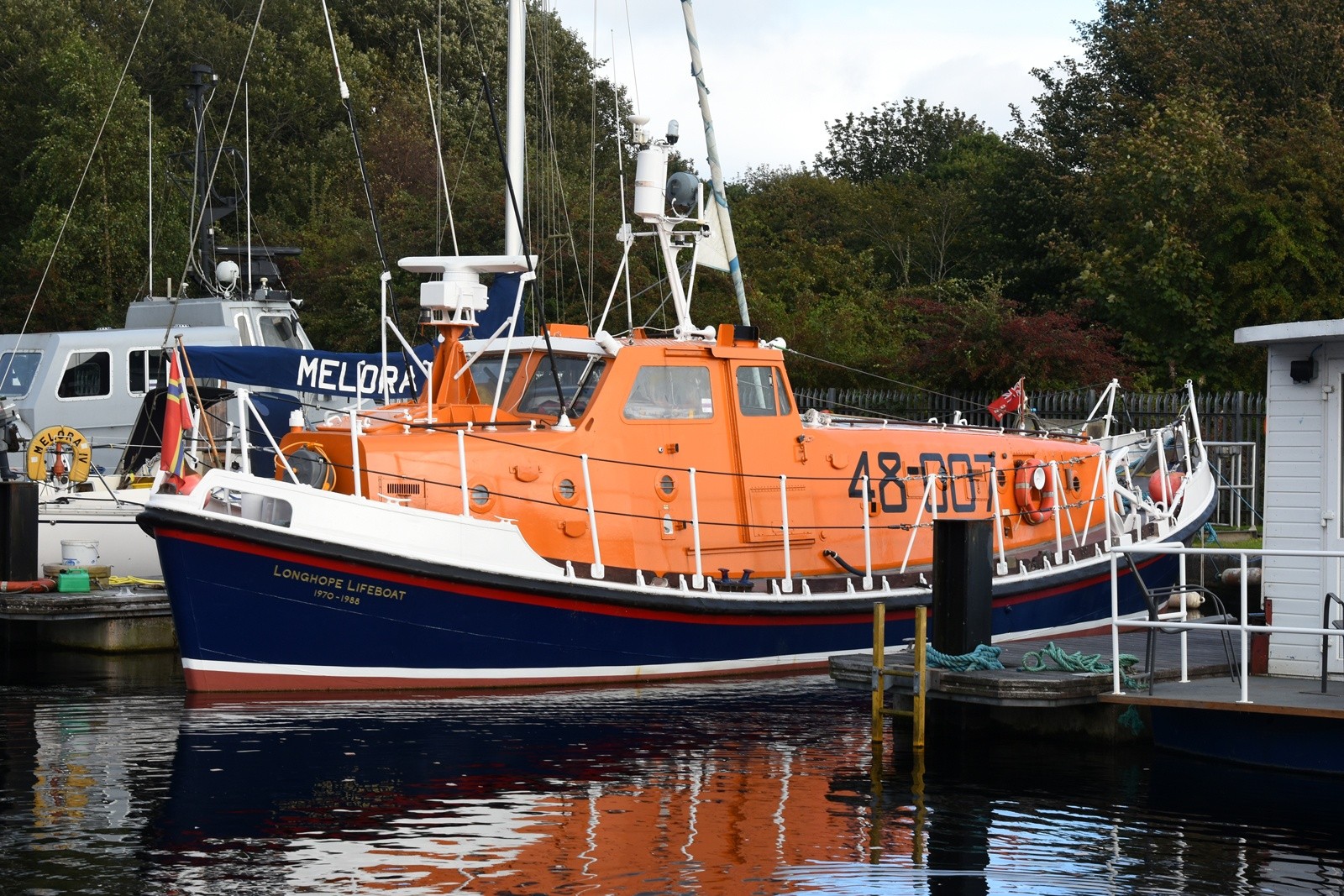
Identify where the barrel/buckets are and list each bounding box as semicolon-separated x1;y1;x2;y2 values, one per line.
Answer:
61;540;101;566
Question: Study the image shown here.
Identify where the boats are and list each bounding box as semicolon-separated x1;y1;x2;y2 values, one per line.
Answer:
0;1;1245;719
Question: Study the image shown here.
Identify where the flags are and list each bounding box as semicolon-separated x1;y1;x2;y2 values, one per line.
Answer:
160;344;195;497
988;378;1021;421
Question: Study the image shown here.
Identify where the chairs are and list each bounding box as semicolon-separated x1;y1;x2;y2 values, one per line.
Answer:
1320;592;1344;694
1123;551;1242;696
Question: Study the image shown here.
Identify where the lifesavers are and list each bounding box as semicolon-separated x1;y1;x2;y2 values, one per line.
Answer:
26;423;91;483
1013;458;1055;526
